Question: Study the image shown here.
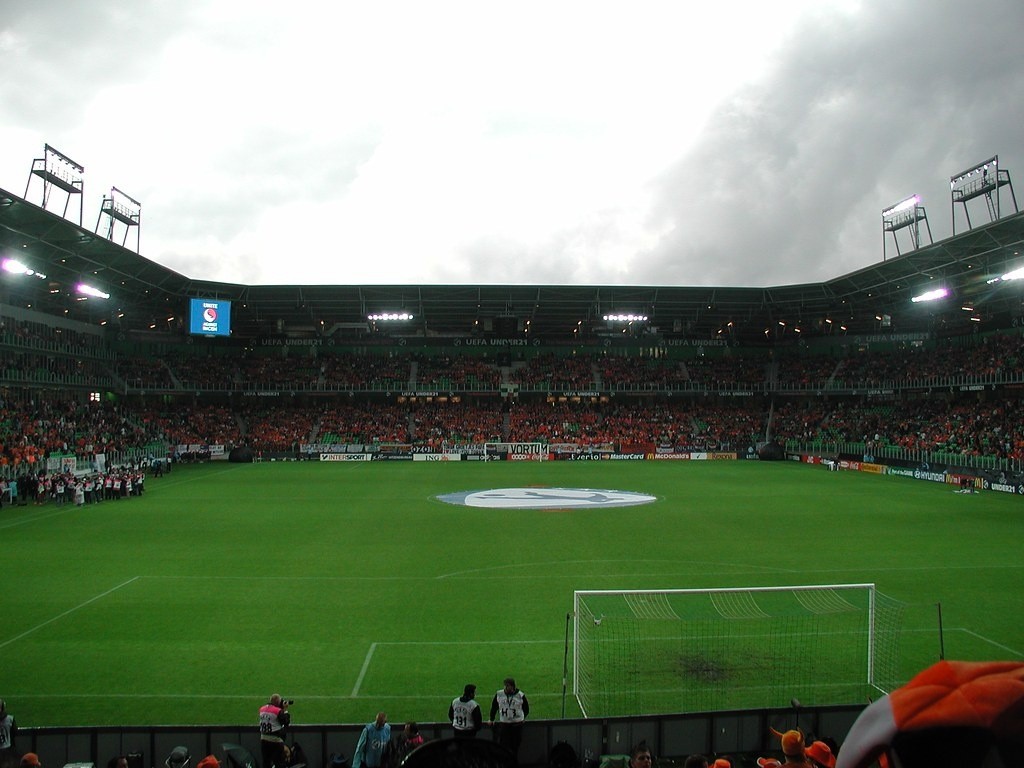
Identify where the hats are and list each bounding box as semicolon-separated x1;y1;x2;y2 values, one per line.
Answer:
20;753;41;768
769;726;805;755
837;660;1024;768
805;740;836;768
757;757;781;768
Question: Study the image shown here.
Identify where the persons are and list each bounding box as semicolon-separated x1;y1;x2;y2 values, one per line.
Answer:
0;693;889;767
449;684;483;767
0;313;1023;509
487;678;529;767
